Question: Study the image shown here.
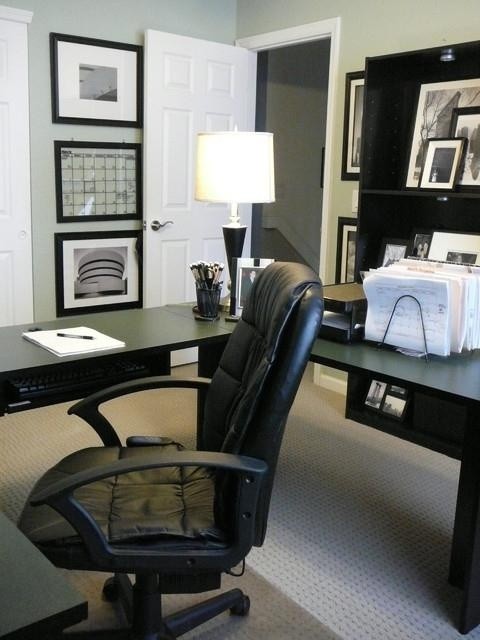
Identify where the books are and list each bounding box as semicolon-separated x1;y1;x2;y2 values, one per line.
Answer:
22;326;127;358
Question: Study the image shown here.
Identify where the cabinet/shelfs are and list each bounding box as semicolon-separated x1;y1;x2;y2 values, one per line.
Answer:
353;40;480;284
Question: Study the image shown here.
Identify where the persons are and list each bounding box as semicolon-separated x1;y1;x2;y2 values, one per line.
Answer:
241;270;256;305
413;241;429;258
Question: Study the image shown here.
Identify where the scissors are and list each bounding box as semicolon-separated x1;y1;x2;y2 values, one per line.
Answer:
204;267;214;289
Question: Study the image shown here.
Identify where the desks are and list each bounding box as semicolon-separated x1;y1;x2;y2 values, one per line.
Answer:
0;304;480;640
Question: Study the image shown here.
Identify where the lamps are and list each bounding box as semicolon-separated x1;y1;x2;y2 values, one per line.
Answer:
194;124;277;322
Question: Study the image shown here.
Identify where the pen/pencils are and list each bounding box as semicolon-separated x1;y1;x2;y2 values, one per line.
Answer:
190;263;223;314
57;334;93;340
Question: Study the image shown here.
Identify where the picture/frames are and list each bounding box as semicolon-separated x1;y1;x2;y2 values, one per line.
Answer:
53;231;143;317
342;70;364;185
419;137;466;191
448;107;479;196
410;227;433;263
53;140;142;222
423;229;480;267
375;238;409;277
399;73;479;194
334;217;356;282
49;32;144;128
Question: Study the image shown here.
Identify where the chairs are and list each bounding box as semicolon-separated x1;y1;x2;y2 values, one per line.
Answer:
18;262;323;640
